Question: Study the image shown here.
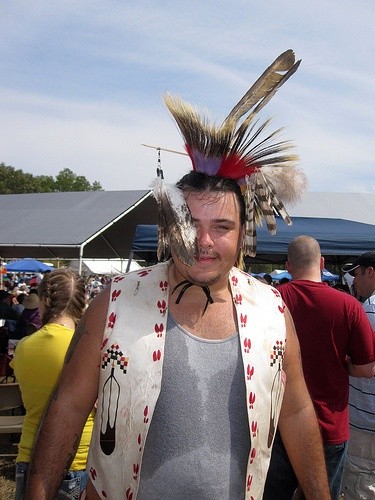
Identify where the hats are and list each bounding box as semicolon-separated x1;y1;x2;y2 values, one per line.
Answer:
341;251;375;272
22;293;39;309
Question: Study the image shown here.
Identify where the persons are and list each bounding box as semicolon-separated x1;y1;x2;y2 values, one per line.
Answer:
1;269;113;382
260;235;374;500
255;274;366;304
24;169;336;498
337;250;374;500
9;268;98;500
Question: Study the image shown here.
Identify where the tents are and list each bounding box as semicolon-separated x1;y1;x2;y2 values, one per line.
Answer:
249;266;339;283
123;215;375;277
2;260;54;278
